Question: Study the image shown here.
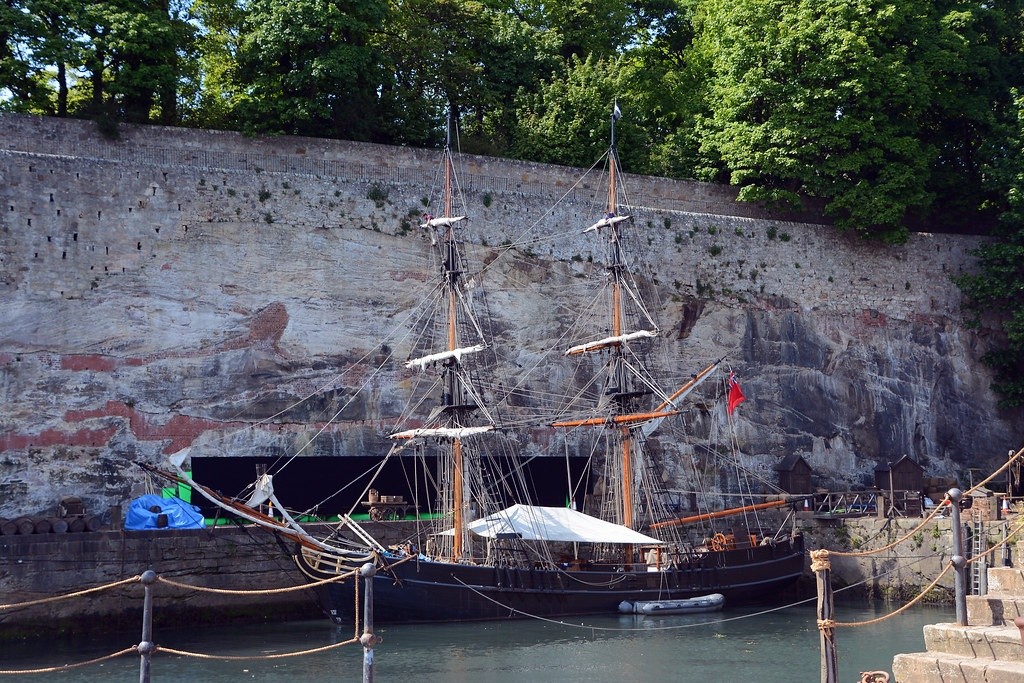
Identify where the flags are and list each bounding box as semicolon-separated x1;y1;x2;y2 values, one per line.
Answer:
728;371;745;414
615;104;621;120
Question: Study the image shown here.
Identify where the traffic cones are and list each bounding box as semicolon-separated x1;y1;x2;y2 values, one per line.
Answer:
1000;495;1010;516
803;499;811;511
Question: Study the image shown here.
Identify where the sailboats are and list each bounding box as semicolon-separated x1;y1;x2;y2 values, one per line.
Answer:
128;96;821;626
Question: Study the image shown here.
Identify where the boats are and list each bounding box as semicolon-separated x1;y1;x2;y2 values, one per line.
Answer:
617;592;727;616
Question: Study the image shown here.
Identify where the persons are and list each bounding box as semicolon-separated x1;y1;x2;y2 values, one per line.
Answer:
422;213;436;231
604;210;618;232
702;530;714;544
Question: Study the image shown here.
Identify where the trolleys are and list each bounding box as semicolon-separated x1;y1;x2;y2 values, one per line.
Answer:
360;500;422;521
